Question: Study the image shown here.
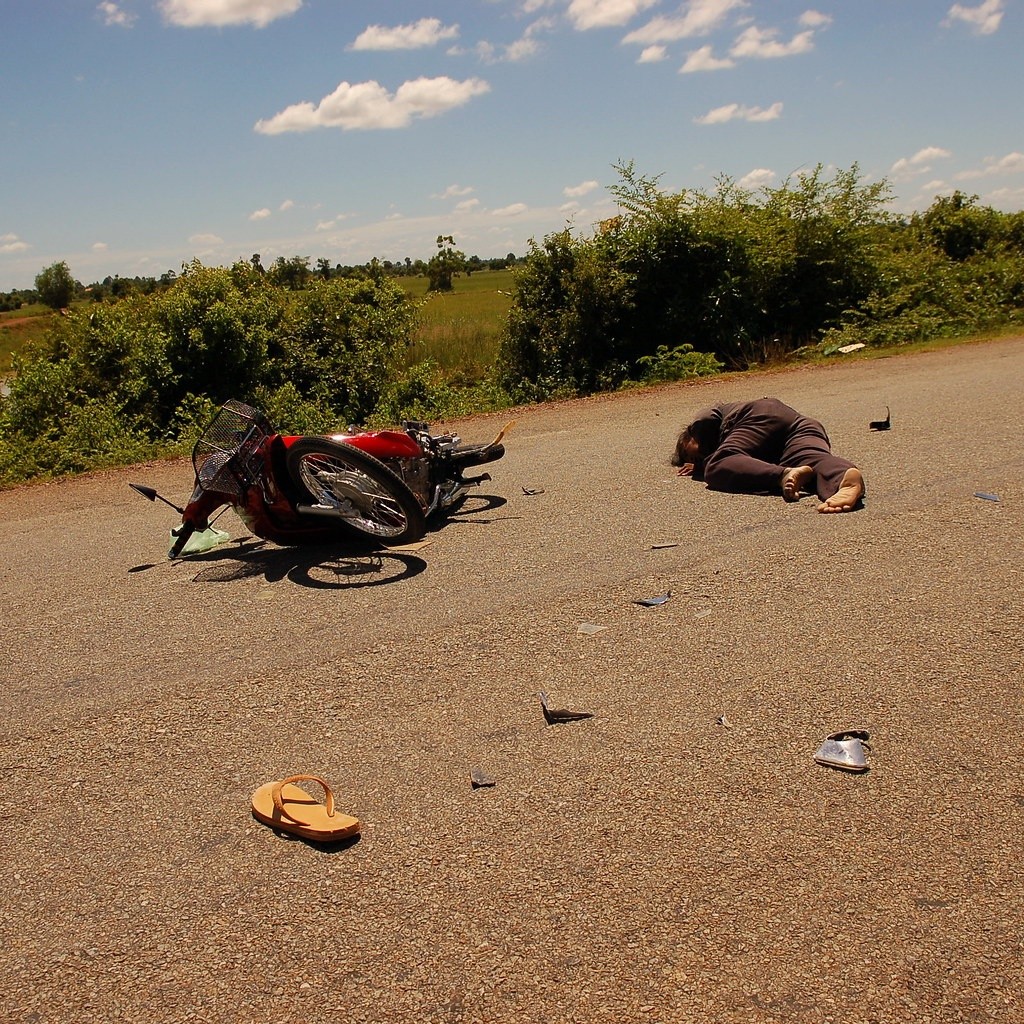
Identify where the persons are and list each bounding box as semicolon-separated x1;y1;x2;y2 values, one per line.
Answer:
672;397;865;512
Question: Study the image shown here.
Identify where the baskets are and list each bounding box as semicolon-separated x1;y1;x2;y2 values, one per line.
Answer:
192;399;276;499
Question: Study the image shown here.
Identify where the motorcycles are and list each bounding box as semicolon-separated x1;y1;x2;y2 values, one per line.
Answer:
128;398;517;561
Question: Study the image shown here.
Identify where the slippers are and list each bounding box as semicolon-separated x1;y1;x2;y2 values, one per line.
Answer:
251;775;360;839
